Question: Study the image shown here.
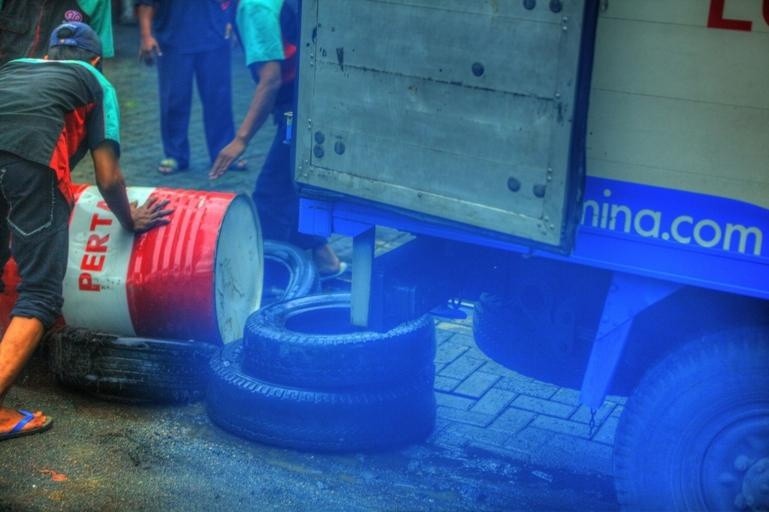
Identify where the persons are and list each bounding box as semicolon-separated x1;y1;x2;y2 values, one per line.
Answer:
210;2;348;286
0;19;177;438
2;0;116;67
133;2;249;179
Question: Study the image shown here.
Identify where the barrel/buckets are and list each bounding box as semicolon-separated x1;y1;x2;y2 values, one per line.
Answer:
0;181;268;348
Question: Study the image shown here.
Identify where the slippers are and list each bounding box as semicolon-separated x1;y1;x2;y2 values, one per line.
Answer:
229;160;248;170
158;158;180;176
0;407;55;439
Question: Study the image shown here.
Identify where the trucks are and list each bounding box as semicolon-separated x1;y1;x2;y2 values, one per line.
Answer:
282;0;769;512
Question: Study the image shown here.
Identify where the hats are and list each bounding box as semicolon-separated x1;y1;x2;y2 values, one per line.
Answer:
48;22;104;72
